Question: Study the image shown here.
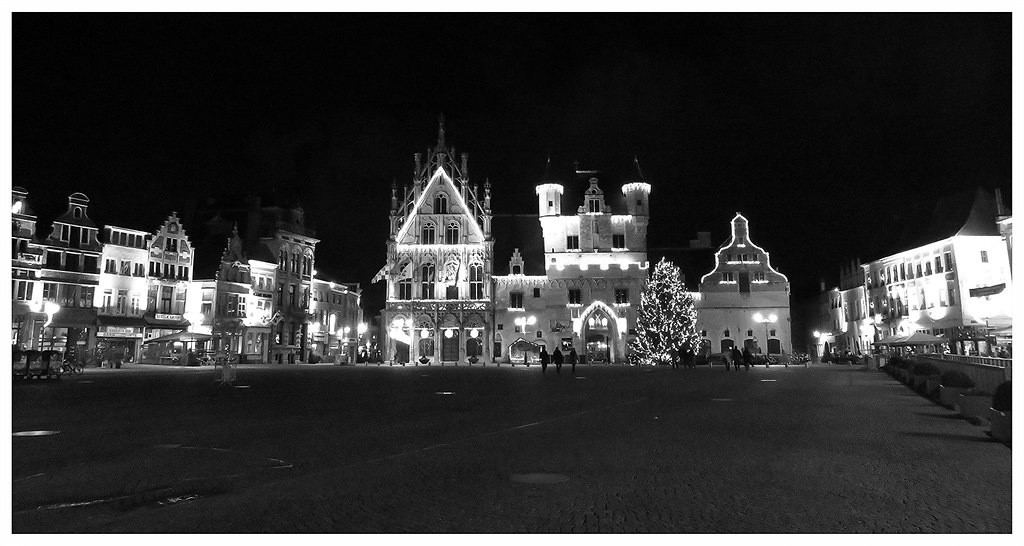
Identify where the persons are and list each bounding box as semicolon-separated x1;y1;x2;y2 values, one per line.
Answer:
539;346;549;373
733;346;743;372
552;346;565;373
743;346;752;372
569;348;579;373
669;343;698;371
723;346;732;371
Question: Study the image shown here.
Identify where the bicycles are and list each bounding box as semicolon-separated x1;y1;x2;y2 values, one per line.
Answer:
57;358;84;376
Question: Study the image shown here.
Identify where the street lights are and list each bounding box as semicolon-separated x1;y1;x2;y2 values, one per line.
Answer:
752;311;779;358
513;315;537;365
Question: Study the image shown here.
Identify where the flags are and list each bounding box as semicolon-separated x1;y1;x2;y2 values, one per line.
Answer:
391;261;413;283
371;263;390;284
